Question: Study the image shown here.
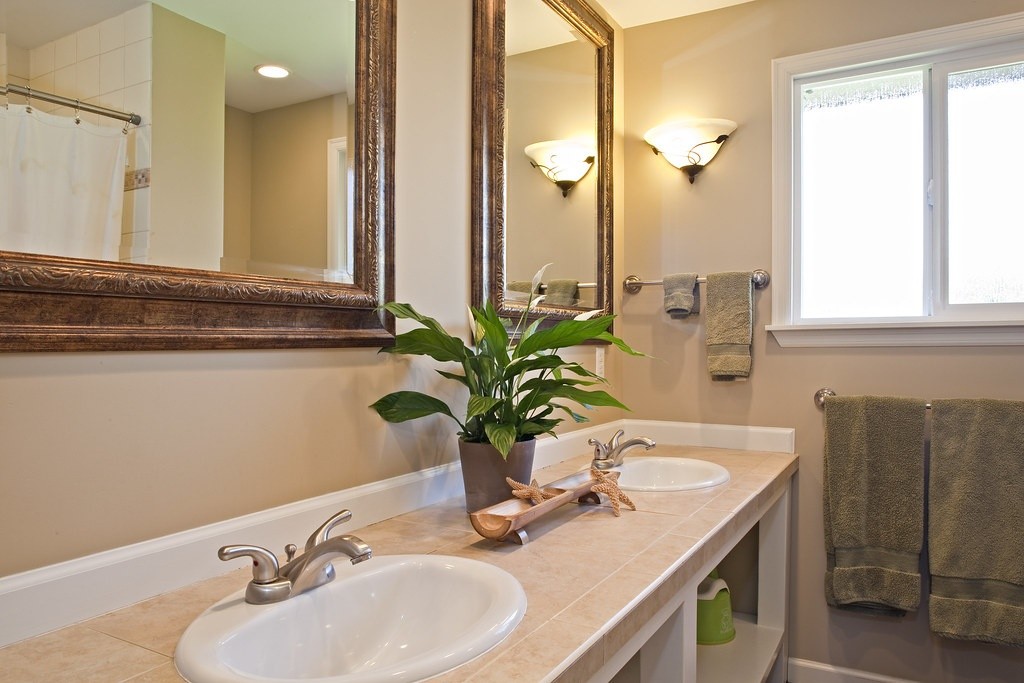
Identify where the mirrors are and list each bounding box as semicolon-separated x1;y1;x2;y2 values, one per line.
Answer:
471;0;613;345
0;0;397;350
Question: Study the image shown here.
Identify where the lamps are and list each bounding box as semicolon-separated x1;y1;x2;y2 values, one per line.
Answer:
254;65;292;79
525;139;595;198
643;118;737;184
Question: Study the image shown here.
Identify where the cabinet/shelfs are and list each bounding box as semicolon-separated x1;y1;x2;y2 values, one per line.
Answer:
584;477;792;683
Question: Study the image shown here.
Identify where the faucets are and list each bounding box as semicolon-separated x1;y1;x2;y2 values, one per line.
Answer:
588;429;656;470
217;510;374;605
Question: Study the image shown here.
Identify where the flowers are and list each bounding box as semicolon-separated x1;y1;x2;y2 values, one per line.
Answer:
369;264;668;463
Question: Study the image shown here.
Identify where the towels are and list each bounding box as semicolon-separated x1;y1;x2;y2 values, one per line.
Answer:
663;272;701;319
705;271;758;382
543;279;580;307
506;281;543;306
823;397;927;619
927;397;1023;649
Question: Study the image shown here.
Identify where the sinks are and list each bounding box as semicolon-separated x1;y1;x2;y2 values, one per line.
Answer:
174;553;530;682
596;456;731;492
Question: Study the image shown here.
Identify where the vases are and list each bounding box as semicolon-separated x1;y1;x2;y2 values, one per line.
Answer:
459;434;534;514
